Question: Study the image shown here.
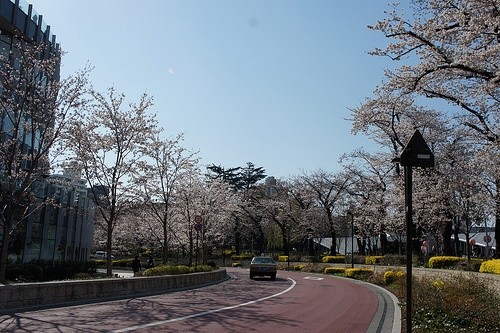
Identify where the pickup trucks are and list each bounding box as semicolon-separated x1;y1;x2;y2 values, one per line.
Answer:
249;257;277;280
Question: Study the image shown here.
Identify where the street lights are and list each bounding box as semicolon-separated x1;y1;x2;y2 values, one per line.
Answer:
346;210;355;268
